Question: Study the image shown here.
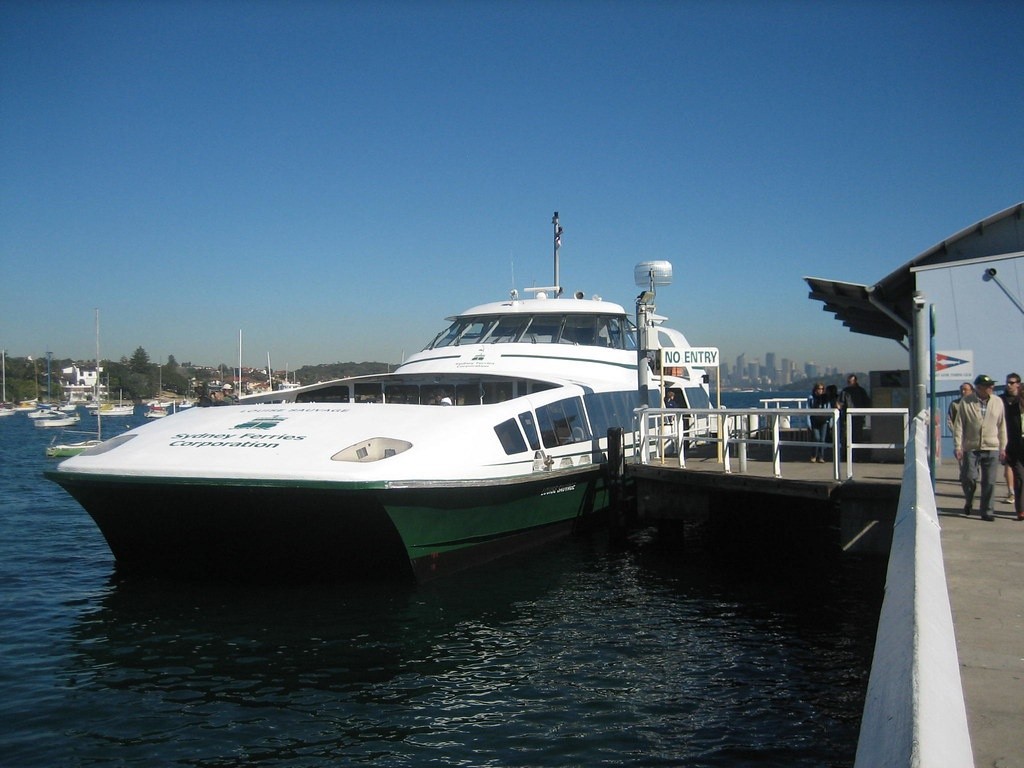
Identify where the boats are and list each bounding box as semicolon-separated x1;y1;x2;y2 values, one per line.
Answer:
0;308;194;457
41;212;760;585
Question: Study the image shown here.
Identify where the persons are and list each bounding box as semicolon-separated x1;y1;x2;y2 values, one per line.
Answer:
999;373;1021;503
947;383;979;480
664;392;677;420
1007;383;1024;519
810;382;838;462
953;375;1007;521
836;374;868;461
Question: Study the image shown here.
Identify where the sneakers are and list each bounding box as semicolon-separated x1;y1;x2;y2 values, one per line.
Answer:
1005;492;1015;504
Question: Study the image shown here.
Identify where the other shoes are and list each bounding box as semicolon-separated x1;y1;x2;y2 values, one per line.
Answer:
811;456;816;463
819;458;825;463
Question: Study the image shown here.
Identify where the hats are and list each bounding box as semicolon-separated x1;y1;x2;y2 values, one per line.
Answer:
974;375;998;385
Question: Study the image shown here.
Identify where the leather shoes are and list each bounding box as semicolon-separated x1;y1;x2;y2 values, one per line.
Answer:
1018;512;1024;520
982;515;994;521
964;504;972;515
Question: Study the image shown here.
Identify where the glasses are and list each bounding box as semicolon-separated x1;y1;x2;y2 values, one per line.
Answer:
817;388;824;390
1007;381;1018;385
